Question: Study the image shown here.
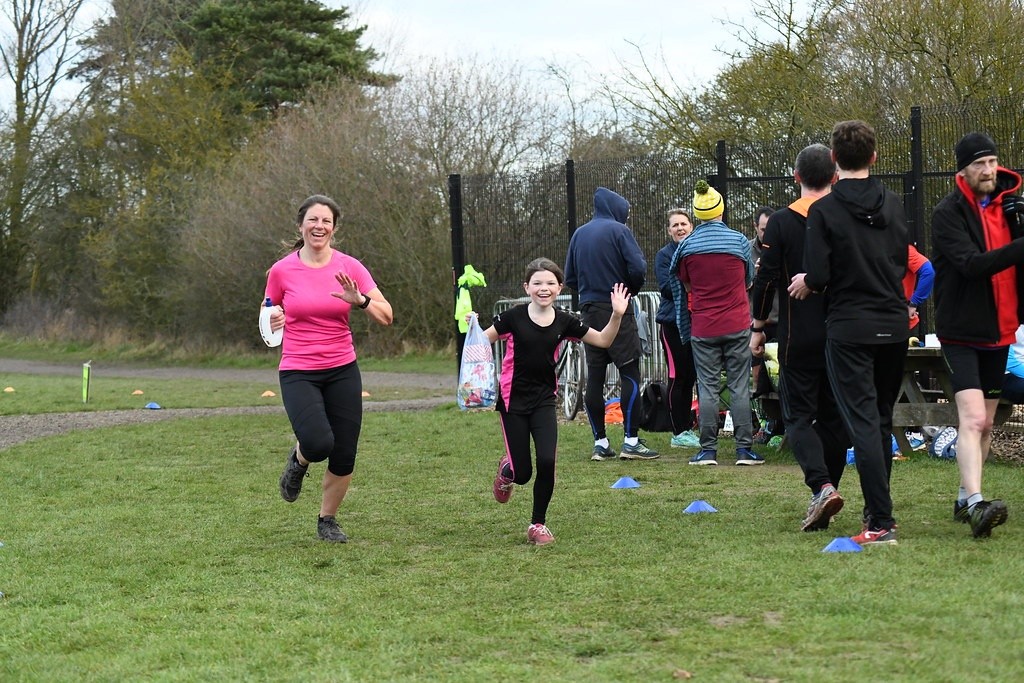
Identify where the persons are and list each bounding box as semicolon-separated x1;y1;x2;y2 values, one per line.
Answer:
259;194;394;544
928;131;1024;538
748;205;782;395
654;208;702;448
748;143;851;529
564;187;661;461
464;257;631;545
669;179;766;467
902;244;935;450
786;119;908;540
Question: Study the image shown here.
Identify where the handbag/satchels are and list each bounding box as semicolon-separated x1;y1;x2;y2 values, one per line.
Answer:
639;378;676;433
456;310;499;413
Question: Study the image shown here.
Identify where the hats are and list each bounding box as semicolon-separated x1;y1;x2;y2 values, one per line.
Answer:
691;180;726;220
954;133;997;172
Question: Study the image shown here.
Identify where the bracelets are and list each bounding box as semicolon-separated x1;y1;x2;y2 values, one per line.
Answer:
909;300;917;307
750;321;766;333
358;293;371;309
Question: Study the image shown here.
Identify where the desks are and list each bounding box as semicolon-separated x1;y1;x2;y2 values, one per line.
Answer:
892;347;1012;456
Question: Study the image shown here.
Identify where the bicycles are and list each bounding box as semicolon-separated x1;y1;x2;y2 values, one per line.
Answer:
554;305;585;422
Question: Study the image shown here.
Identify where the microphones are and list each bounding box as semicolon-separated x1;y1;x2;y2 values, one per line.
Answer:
908;337;924;347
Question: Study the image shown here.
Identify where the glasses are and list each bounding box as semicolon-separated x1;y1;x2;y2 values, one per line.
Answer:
970;160;998;169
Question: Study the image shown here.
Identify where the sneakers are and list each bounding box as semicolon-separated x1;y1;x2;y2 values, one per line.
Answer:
526;523;556;546
968;500;1008;538
735;447;765;465
277;448;308;503
316;515;348;543
492;456;513;504
849;525;899;546
953;499;972;523
688;448;718;465
591;437;617;460
907;437;927;452
753;427;781;444
670;429;702;449
801;482;845;531
619;437;660;460
